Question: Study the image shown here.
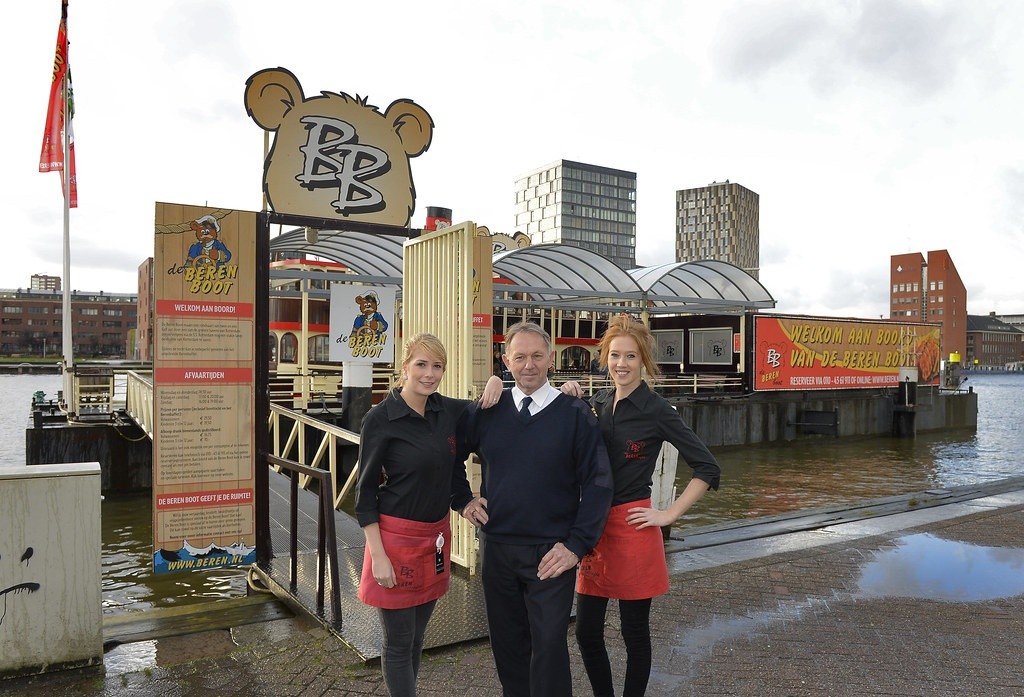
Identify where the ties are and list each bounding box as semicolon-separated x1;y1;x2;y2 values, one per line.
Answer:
519;397;533;416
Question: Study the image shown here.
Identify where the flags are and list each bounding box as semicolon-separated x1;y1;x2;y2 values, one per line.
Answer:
39;18;78;211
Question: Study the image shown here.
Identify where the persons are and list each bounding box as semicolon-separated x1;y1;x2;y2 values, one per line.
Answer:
452;322;613;697
559;313;722;697
353;333;504;697
492;351;502;379
591;351;608;375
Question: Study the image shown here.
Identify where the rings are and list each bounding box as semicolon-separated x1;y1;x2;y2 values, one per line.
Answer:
568;384;574;388
494;398;497;401
377;582;382;586
471;511;475;517
